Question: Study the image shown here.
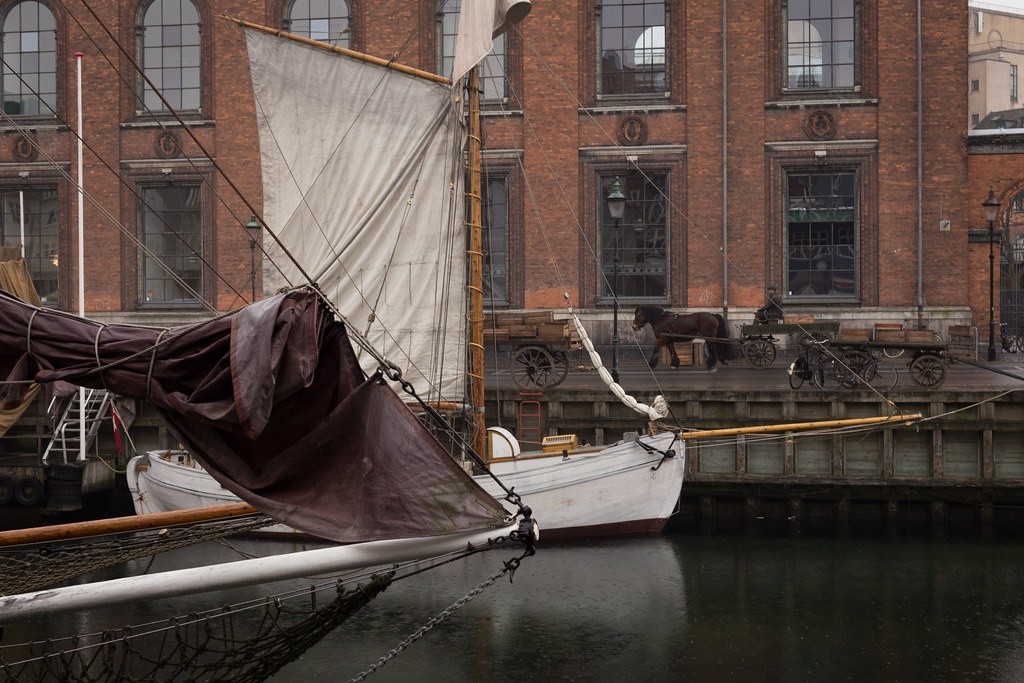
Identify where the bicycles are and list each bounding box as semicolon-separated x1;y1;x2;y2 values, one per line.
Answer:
815;344;898;394
1001;322;1024;354
787;339;830;389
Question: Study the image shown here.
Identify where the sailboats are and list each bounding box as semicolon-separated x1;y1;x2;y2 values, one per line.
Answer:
126;0;687;539
0;0;544;683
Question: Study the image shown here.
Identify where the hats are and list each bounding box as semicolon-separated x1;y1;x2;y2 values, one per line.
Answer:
767;286;777;290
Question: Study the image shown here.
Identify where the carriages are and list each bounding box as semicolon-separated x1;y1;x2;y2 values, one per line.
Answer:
632;304;840;371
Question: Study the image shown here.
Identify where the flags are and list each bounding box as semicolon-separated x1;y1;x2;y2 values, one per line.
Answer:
110;399;124;456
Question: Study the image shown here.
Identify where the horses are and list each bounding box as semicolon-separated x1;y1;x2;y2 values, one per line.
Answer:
632;302;729;373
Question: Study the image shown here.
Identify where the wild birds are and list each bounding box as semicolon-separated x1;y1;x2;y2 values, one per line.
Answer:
651;395;669;427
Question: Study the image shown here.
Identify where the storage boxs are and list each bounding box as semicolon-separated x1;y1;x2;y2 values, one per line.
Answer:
497;325;539;337
952;354;975;361
948;325;975;337
483;325;498;329
554;319;582;349
873;323;902;330
840;329;873;342
483;329;510;341
948;344;975;355
875;329;905;343
783;315;815;324
523;311;554;325
482;314;496;325
537;323;570;338
663;342;704;366
496;313;524;325
951;335;976;345
905;329;934;343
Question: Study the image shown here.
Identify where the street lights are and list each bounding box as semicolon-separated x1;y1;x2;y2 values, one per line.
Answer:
245;214;261;302
980;182;1001;363
605;176;629;396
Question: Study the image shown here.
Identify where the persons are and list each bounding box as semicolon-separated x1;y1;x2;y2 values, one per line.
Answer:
753;286;783;326
